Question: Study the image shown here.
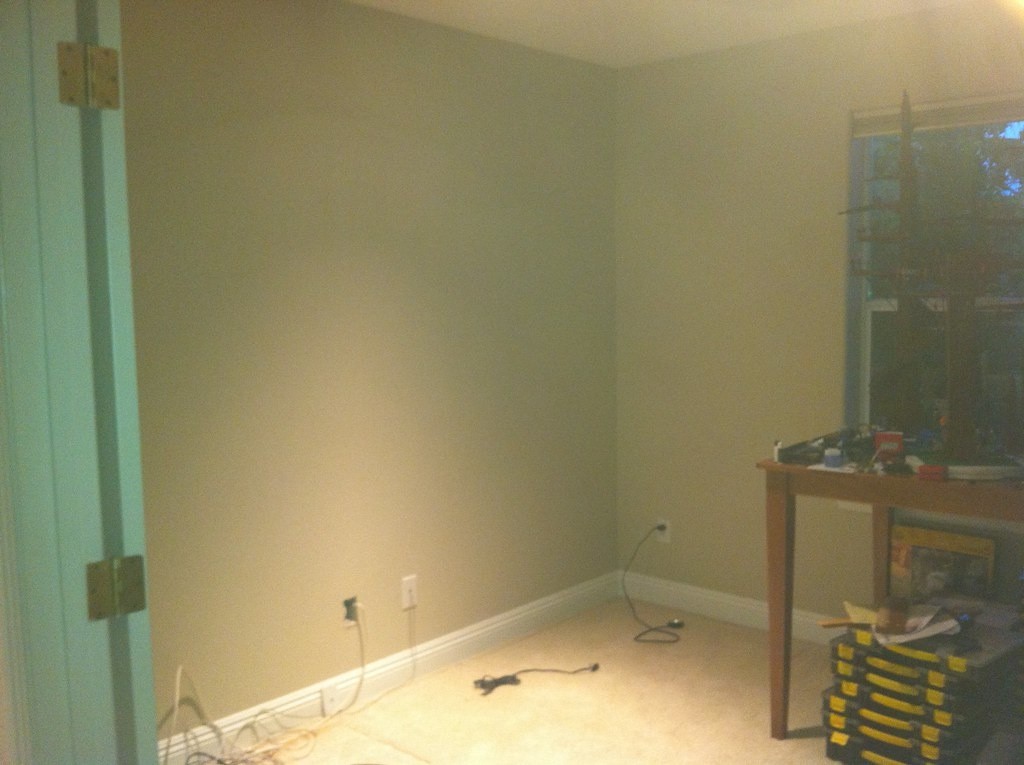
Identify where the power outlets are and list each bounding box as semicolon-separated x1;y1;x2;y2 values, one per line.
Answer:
657;518;672;543
401;575;418;610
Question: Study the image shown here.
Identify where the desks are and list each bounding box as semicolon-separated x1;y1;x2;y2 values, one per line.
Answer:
755;456;1024;740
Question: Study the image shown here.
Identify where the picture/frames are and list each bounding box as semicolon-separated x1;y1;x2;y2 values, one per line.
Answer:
885;519;1000;608
